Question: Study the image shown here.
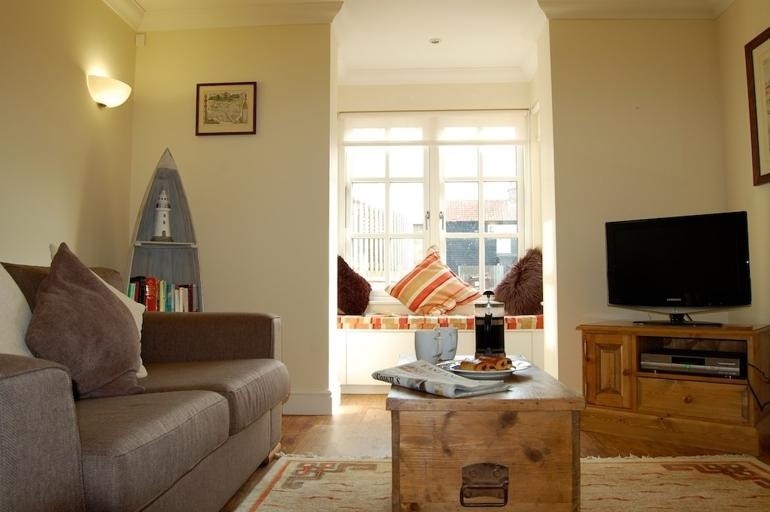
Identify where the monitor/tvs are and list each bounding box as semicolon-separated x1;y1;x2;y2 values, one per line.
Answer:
605;211;752;327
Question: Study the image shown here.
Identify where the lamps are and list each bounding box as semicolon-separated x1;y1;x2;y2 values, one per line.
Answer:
87;76;132;108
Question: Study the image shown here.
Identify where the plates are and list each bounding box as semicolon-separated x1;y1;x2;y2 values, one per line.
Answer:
448;357;534;379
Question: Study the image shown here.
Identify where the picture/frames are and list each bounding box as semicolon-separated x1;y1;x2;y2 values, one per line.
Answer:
195;81;257;136
744;26;770;186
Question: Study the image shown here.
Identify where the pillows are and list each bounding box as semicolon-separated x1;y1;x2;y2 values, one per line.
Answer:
50;243;149;378
495;248;543;316
337;255;371;315
25;242;146;396
385;245;482;316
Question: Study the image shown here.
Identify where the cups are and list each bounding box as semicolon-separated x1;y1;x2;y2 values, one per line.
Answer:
414;330;444;366
439;327;459;361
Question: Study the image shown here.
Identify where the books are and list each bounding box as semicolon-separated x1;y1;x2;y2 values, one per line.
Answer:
129;277;201;313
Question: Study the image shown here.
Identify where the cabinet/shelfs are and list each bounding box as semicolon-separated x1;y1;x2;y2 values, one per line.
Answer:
125;148;205;312
576;320;770;456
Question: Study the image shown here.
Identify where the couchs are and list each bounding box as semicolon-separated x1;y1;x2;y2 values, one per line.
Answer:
0;311;291;512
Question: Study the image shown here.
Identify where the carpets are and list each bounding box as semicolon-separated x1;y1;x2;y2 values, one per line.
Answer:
234;452;770;512
